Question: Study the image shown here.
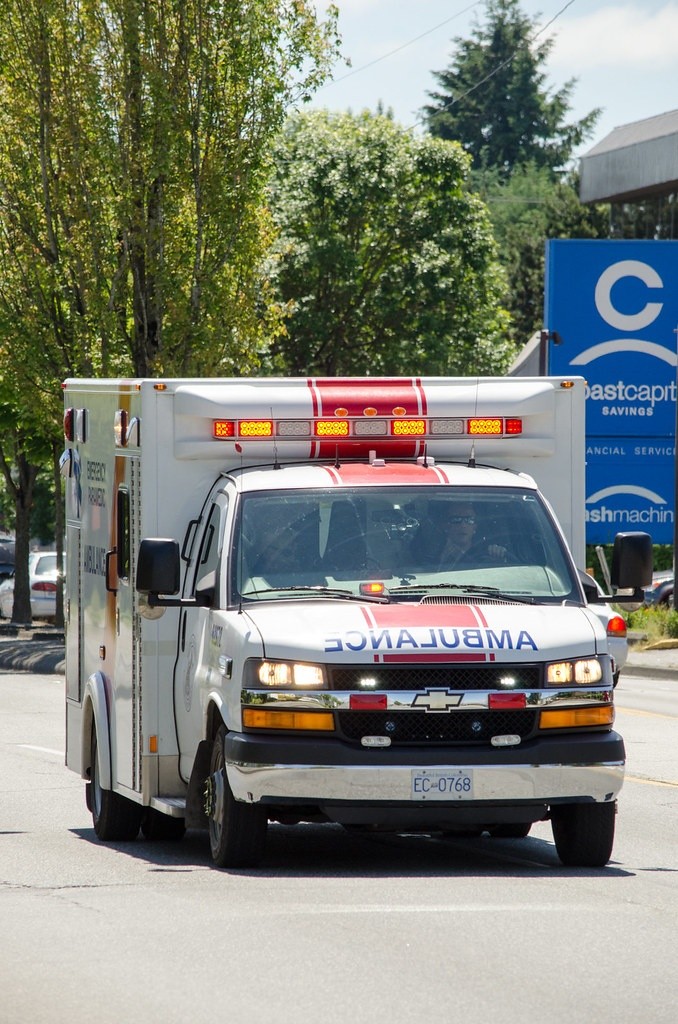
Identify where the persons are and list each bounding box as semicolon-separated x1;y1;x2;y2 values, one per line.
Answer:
413;500;508;572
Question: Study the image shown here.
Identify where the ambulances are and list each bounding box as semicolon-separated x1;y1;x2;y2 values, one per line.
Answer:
58;372;654;871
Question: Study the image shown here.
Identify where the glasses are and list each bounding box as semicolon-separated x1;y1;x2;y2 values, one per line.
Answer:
448;515;476;525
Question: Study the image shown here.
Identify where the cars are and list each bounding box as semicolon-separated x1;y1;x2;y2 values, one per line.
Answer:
0;532;68;624
643;574;674;611
